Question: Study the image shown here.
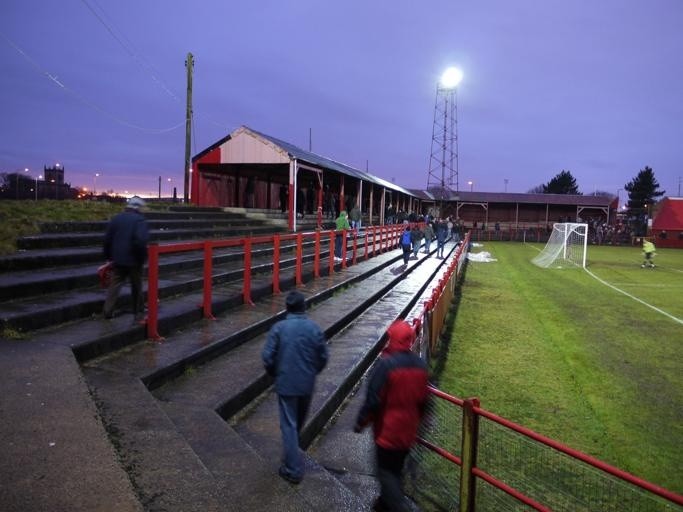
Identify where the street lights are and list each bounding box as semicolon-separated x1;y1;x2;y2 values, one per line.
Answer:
167;177;172;198
616;188;623;208
422;66;459;193
502;178;508;193
35;175;42;200
93;173;100;195
468;181;473;192
15;166;28;200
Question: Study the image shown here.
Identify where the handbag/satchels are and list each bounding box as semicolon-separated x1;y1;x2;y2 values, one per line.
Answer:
98;263;112;289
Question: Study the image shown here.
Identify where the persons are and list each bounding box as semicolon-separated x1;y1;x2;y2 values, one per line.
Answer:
355;321;433;510
101;196;151;320
547;213;667;245
386;207;501;265
279;182;361;262
641;237;656;268
262;289;332;483
